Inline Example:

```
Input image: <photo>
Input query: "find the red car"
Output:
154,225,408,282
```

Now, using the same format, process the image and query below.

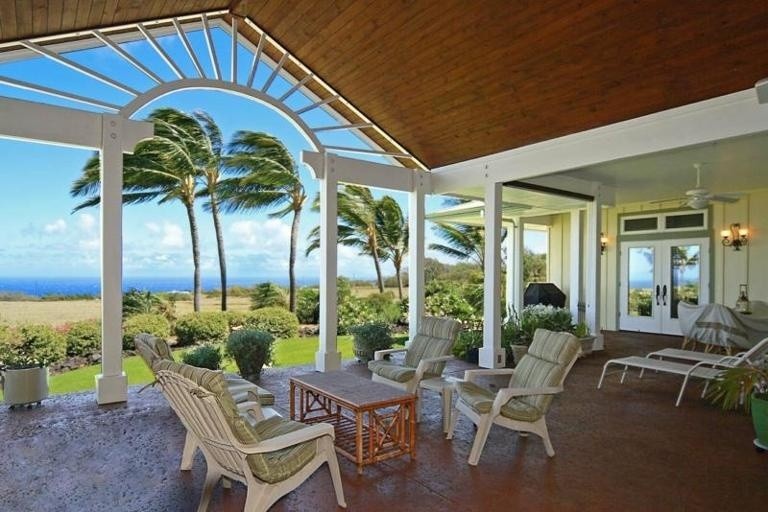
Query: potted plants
2,325,51,410
459,331,483,364
347,319,390,364
701,364,768,454
223,330,273,382
575,324,595,355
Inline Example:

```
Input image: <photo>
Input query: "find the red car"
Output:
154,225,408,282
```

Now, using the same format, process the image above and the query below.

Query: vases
510,345,528,365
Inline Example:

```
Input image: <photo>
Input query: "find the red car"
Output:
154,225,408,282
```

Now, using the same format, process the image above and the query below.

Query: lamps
600,233,607,255
686,199,705,209
720,223,749,251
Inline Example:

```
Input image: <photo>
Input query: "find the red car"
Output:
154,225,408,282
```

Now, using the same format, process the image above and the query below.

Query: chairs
597,336,768,408
446,327,583,466
677,301,732,355
133,332,348,512
367,316,462,433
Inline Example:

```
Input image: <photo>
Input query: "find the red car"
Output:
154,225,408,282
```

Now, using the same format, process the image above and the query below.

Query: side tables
416,374,463,433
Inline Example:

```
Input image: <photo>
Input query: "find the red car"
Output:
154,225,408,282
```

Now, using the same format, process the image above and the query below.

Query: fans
649,163,740,209
